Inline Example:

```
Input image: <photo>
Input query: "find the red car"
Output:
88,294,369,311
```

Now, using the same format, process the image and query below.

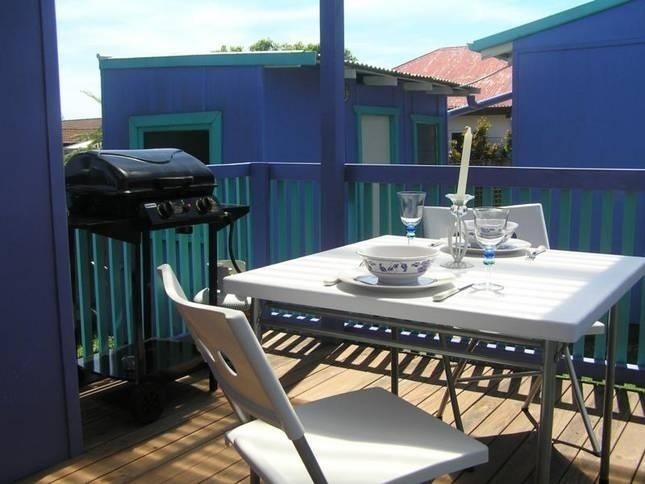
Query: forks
524,245,546,266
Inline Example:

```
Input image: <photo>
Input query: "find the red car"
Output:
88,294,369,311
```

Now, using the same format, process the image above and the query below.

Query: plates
338,268,457,294
446,236,530,255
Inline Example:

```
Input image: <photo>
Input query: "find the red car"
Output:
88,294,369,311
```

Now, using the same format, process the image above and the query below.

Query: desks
68,204,250,425
222,234,645,484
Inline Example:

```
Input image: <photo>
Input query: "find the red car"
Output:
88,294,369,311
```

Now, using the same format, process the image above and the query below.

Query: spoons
323,259,370,287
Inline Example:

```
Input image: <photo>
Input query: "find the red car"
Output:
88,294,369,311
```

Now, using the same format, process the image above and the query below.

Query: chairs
417,204,609,456
157,254,492,484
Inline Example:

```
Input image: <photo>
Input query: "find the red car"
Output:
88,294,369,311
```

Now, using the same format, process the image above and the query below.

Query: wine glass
396,190,427,248
474,206,510,292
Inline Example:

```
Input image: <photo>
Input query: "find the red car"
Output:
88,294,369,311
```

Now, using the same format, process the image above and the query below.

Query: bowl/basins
454,220,519,246
353,245,440,283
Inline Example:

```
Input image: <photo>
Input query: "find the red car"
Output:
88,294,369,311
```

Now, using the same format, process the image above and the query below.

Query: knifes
429,280,479,302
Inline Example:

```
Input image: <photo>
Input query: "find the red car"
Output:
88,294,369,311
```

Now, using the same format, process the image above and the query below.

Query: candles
458,121,474,194
